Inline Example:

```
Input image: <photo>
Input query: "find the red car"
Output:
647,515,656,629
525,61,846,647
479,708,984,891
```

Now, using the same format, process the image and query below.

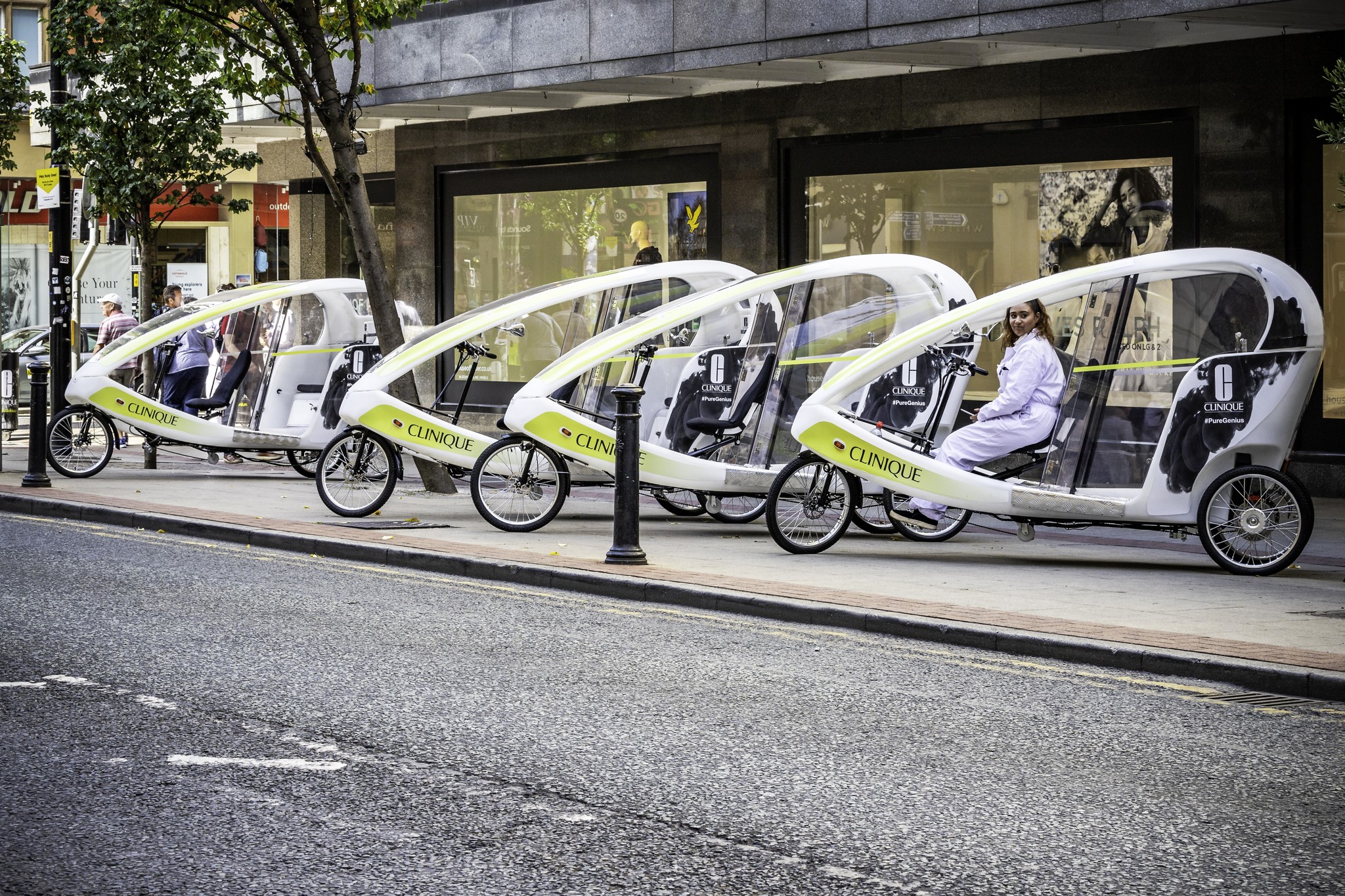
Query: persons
614,220,663,387
1042,167,1173,396
887,297,1064,530
92,292,142,448
459,290,597,385
150,283,297,463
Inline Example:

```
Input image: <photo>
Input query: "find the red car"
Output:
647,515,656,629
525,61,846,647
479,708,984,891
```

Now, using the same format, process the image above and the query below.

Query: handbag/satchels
215,335,225,355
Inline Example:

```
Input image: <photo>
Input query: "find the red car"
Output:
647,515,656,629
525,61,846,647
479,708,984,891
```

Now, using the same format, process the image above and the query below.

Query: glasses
1044,261,1060,275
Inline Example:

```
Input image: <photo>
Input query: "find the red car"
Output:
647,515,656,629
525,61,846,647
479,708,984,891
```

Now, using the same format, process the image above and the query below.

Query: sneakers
122,436,128,445
890,508,938,531
113,438,126,448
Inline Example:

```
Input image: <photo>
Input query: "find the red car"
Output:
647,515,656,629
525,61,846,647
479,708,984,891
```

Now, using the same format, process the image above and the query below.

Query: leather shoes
256,451,284,460
223,454,243,463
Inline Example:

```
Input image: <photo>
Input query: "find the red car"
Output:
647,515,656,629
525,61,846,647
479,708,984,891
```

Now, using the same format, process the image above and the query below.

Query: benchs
297,382,325,394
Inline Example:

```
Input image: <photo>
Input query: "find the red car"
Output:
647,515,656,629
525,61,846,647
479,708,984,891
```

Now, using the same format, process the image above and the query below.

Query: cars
1,322,106,406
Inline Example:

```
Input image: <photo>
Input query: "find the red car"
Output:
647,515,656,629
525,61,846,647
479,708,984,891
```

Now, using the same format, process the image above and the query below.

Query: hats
96,293,122,306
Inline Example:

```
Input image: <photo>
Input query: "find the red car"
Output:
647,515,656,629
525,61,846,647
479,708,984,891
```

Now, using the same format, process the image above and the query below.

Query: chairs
1005,358,1101,464
185,348,252,418
685,350,777,444
496,369,581,432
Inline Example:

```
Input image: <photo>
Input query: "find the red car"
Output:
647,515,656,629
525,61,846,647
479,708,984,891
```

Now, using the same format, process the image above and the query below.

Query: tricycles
471,251,982,541
763,245,1326,576
312,257,784,520
47,275,422,484
